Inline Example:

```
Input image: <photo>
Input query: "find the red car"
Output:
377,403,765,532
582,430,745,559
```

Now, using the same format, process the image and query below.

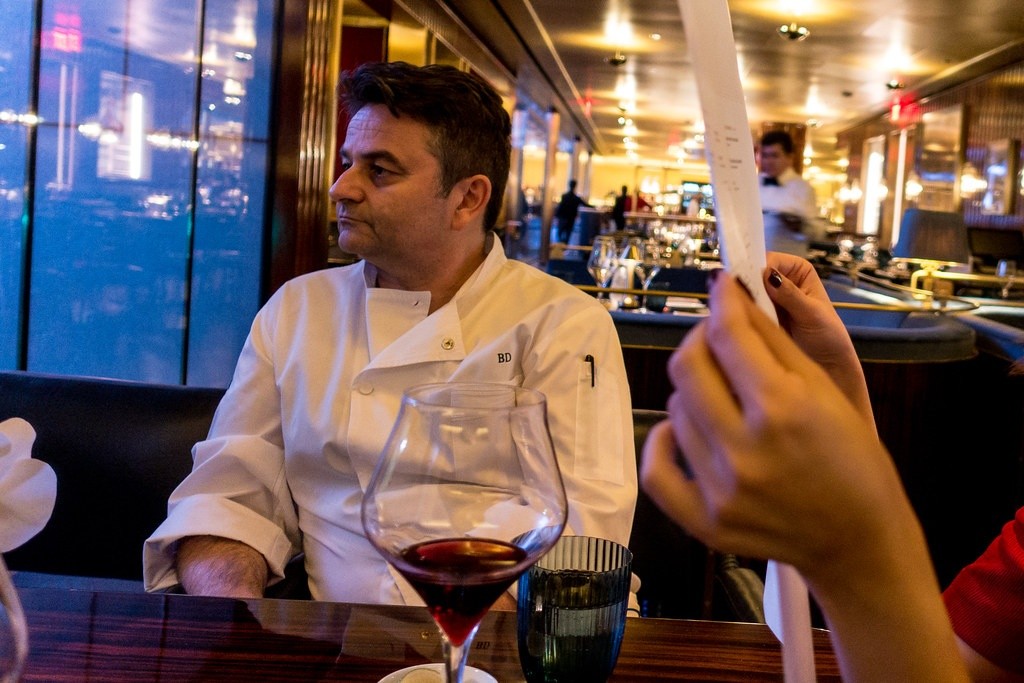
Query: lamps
893,207,970,296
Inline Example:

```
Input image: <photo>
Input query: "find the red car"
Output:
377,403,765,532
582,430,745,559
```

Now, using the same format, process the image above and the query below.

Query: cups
517,535,632,683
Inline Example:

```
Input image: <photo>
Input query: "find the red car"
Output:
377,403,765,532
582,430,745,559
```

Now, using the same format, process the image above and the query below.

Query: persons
757,131,818,259
555,180,595,245
143,61,640,618
640,252,1024,683
612,186,652,230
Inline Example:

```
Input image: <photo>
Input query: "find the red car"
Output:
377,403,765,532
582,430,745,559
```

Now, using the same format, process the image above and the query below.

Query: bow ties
763,175,779,186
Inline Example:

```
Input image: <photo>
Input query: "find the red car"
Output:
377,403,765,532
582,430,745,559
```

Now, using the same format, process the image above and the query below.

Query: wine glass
587,236,618,300
362,382,570,683
996,261,1015,297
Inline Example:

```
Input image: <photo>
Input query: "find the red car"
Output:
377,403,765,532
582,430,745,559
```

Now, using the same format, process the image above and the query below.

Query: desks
0,571,845,681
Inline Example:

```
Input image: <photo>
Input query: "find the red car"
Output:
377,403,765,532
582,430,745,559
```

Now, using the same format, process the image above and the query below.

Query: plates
373,662,496,683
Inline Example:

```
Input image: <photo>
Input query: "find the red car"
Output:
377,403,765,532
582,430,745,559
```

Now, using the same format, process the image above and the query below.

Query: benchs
551,212,1024,623
